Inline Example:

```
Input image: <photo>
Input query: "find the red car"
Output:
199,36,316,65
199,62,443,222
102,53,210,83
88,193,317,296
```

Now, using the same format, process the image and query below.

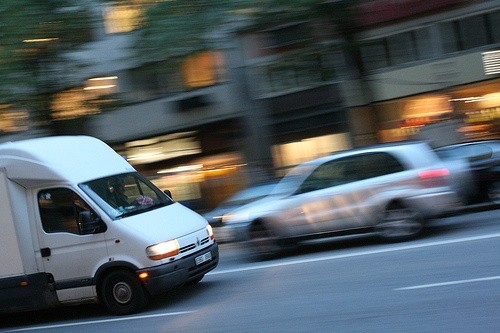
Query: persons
109,179,131,211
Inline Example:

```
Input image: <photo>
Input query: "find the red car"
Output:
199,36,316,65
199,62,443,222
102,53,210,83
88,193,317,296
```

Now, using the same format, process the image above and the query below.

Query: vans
0,134,220,315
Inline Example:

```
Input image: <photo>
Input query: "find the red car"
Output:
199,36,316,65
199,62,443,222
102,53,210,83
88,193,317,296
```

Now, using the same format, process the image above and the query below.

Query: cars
224,141,469,258
201,174,298,244
429,136,500,218
